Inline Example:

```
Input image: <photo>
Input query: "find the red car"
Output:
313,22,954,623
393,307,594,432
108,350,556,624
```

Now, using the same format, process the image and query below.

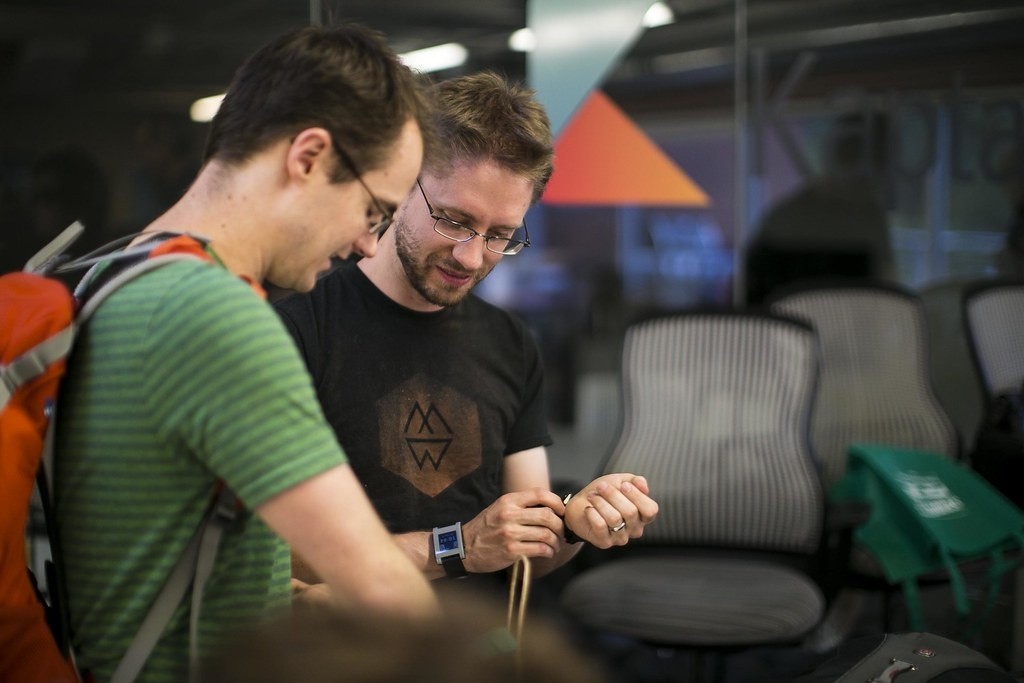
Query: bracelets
560,492,587,544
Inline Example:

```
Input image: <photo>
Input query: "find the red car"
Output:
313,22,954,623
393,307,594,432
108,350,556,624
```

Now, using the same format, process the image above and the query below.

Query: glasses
328,134,392,231
416,178,531,256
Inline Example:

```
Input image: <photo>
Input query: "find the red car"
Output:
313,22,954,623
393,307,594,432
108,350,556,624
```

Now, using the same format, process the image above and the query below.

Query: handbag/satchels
839,439,1024,635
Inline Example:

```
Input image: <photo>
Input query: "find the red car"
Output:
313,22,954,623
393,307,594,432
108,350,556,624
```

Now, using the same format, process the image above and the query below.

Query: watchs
431,520,478,576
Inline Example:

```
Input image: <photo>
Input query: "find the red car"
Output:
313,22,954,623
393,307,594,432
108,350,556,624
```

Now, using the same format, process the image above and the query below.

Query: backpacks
0,222,267,682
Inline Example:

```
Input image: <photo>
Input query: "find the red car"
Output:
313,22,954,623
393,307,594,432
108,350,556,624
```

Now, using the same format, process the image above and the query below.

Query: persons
270,66,660,683
51,23,445,683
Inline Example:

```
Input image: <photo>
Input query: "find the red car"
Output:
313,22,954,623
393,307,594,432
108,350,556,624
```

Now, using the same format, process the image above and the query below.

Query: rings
609,521,626,532
